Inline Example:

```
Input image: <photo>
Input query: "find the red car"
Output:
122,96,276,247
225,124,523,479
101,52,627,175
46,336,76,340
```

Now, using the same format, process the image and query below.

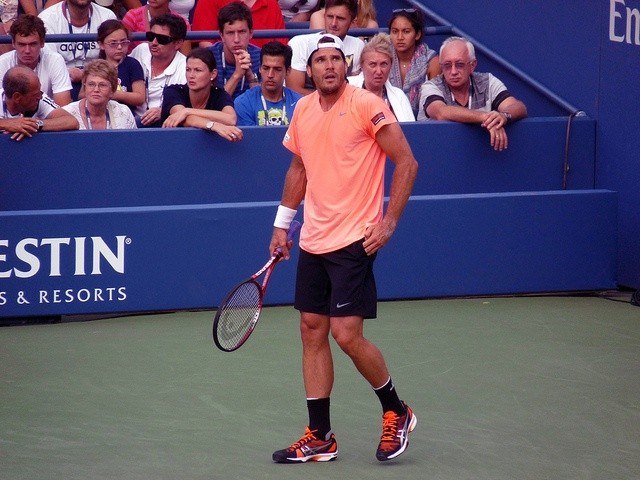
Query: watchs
36,119,44,131
503,111,511,122
206,121,214,130
248,72,259,83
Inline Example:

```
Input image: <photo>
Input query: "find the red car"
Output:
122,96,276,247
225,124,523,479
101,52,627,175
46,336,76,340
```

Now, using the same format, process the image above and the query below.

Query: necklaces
400,63,410,67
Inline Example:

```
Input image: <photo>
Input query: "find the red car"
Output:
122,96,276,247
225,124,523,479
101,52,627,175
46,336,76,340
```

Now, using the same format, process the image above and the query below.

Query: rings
231,133,235,137
496,135,499,138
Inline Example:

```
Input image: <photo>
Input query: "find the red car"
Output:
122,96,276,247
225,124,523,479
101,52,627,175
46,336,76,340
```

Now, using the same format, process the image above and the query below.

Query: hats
305,31,345,64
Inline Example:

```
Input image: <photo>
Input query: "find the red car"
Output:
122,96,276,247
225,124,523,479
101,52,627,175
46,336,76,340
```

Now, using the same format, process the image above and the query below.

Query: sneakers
272,426,338,463
376,400,417,461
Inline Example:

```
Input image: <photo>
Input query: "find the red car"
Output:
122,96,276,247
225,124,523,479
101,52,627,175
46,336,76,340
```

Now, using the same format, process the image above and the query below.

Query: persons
169,0,196,22
78,18,146,116
192,0,289,49
283,0,366,96
388,7,442,121
206,0,261,100
0,0,38,56
278,0,318,21
160,47,244,142
0,64,80,141
43,0,143,19
348,33,416,122
127,13,188,128
417,36,528,150
37,0,117,98
122,0,191,55
310,0,379,44
235,40,304,126
0,13,74,107
269,33,419,465
62,58,138,131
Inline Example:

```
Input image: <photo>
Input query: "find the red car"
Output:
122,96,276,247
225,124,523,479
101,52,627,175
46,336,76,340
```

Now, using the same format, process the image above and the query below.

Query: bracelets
273,205,297,229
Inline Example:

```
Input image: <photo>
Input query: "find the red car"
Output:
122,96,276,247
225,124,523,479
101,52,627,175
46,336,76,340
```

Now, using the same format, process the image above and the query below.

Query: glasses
439,61,473,68
392,8,417,13
103,40,130,48
146,32,178,45
82,82,112,89
